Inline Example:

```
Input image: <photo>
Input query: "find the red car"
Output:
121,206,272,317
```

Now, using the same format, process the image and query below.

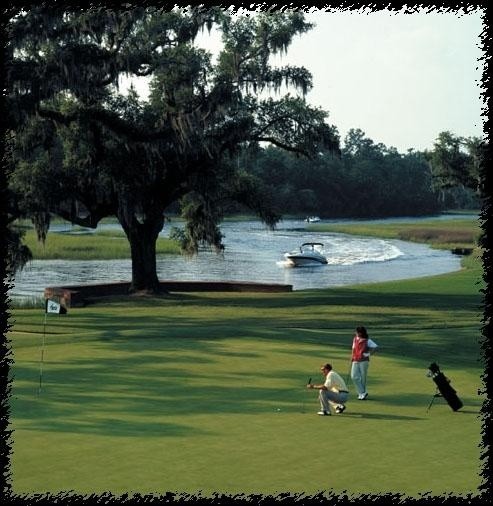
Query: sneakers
358,393,368,400
336,406,344,414
317,411,330,416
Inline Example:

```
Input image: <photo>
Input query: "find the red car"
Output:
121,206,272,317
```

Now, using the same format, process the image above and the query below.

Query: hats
321,364,331,369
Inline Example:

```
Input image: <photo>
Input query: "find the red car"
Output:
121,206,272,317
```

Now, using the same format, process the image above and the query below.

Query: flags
47,300,67,314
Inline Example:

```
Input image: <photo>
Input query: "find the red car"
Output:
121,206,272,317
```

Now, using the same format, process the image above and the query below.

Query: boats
304,215,320,222
285,240,328,265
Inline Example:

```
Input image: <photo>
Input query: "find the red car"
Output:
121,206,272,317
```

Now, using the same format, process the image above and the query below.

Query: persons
351,326,378,400
307,364,349,416
